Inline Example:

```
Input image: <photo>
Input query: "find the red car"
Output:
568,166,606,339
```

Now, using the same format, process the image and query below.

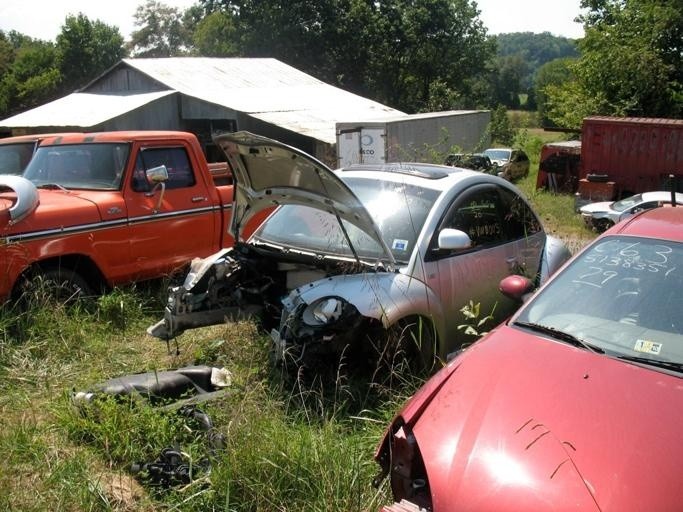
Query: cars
362,193,683,511
0,134,78,178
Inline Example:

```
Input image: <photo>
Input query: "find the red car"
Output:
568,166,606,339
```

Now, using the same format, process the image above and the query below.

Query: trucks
1,125,288,336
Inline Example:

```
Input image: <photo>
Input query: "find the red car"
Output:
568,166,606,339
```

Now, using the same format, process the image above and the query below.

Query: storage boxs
578,178,616,200
